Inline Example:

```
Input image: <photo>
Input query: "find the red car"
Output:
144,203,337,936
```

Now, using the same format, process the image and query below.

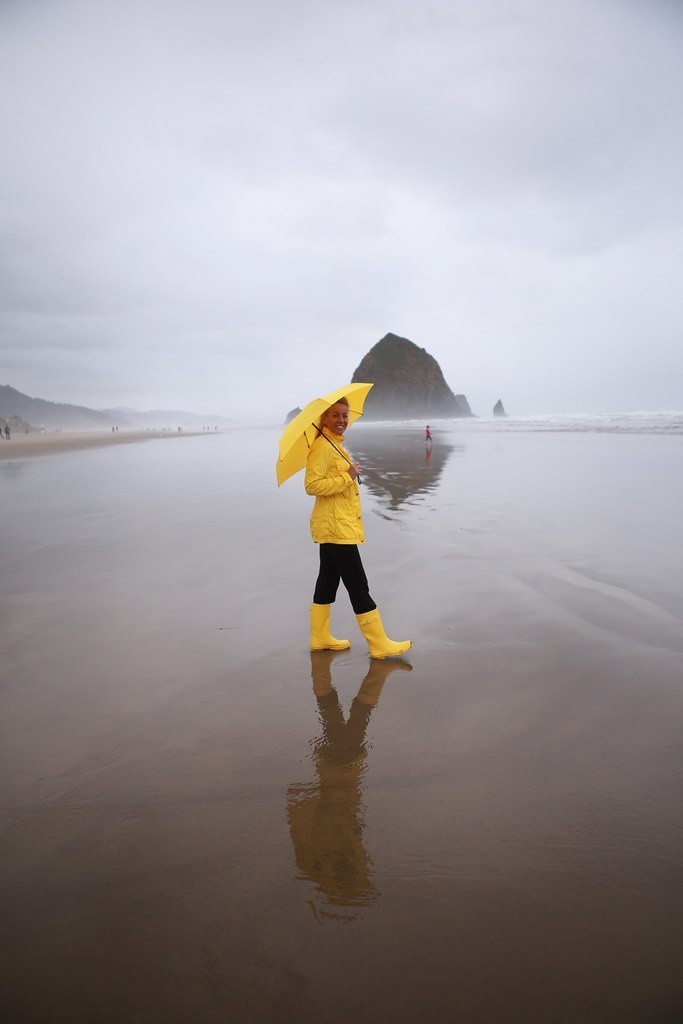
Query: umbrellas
276,383,375,487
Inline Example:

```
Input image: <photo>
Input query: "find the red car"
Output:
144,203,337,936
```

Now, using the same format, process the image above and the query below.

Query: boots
310,648,349,696
309,603,350,651
356,607,412,659
356,659,413,706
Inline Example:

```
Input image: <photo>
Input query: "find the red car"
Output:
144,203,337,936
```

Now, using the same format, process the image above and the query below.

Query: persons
5,424,10,440
0,429,4,439
304,399,414,660
425,426,431,440
